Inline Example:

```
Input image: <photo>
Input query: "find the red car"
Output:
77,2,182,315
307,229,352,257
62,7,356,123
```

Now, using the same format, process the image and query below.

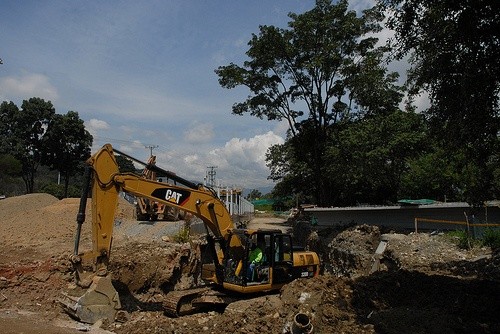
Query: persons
199,184,205,191
158,178,163,182
196,185,199,190
244,243,263,282
191,183,195,188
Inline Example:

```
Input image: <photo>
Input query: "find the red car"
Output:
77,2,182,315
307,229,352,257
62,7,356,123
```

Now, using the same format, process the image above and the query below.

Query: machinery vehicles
54,143,322,326
